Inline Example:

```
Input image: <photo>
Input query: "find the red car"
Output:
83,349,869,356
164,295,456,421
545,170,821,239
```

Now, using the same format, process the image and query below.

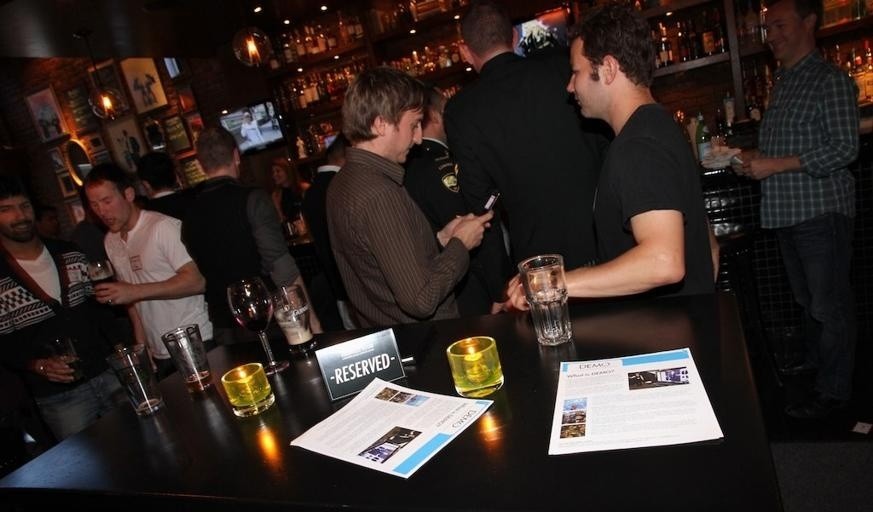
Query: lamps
228,0,274,69
71,32,126,121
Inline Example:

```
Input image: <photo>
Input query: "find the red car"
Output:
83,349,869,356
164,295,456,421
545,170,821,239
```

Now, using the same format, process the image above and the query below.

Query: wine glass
226,278,290,377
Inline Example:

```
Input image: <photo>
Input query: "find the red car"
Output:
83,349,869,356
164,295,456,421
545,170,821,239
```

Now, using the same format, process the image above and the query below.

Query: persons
732,0,861,419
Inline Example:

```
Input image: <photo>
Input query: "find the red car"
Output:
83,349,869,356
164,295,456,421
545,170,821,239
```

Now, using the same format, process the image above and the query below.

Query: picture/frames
117,55,170,118
21,80,113,230
100,113,149,175
84,58,133,115
162,82,209,187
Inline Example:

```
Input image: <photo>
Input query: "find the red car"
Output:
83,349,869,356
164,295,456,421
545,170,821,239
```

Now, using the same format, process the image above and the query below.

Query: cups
85,262,116,293
161,323,211,392
221,363,275,419
268,285,317,354
106,343,162,417
50,338,80,372
446,336,503,399
519,254,571,345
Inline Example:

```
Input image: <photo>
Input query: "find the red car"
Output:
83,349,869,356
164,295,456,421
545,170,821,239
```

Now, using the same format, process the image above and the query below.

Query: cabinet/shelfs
655,2,868,164
261,0,471,165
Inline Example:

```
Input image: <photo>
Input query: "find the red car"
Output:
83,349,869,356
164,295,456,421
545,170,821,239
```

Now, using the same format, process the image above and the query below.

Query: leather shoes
784,389,850,421
779,359,817,379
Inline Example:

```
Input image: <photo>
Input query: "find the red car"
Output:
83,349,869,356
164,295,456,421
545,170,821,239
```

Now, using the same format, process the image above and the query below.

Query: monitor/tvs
216,99,290,158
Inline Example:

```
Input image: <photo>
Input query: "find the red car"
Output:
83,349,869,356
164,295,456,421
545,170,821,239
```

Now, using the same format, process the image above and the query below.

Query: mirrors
140,119,168,155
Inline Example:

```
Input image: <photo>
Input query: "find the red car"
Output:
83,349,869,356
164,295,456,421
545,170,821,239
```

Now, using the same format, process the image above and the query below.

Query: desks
0,292,789,512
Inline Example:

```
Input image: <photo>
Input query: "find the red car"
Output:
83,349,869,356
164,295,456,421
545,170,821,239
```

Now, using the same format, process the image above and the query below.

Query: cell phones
475,190,500,217
730,155,744,165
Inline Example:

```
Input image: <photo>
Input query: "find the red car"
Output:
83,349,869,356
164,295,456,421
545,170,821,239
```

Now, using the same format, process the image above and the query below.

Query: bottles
645,0,873,167
248,2,476,160
